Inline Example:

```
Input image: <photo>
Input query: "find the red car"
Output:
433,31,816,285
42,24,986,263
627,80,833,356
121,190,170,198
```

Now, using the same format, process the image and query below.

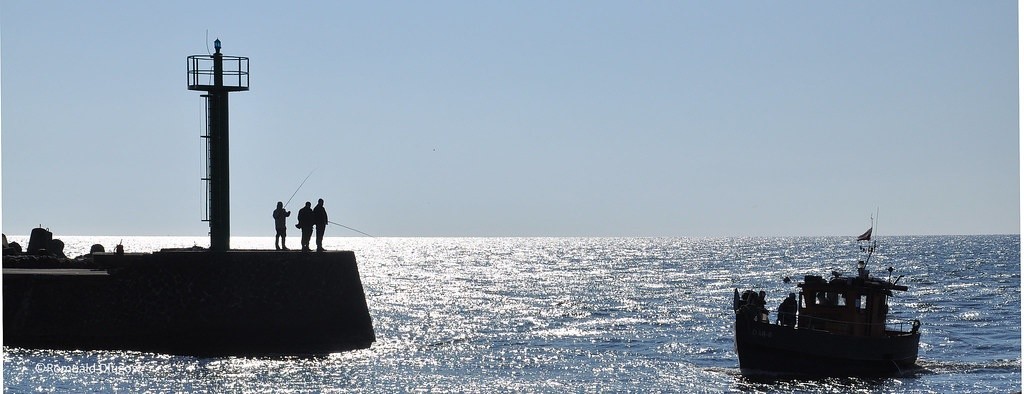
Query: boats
732,244,922,385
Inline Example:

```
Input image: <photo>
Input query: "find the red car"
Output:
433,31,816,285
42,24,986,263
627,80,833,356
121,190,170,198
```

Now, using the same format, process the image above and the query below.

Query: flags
858,227,873,242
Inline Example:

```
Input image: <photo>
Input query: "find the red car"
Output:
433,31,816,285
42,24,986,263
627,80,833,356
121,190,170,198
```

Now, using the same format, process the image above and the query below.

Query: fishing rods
328,221,381,242
283,167,316,209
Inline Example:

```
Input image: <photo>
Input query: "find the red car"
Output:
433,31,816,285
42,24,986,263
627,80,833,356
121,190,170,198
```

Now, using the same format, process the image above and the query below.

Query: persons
778,293,797,329
298,202,313,251
313,199,328,251
816,293,830,305
273,201,290,250
752,290,769,320
